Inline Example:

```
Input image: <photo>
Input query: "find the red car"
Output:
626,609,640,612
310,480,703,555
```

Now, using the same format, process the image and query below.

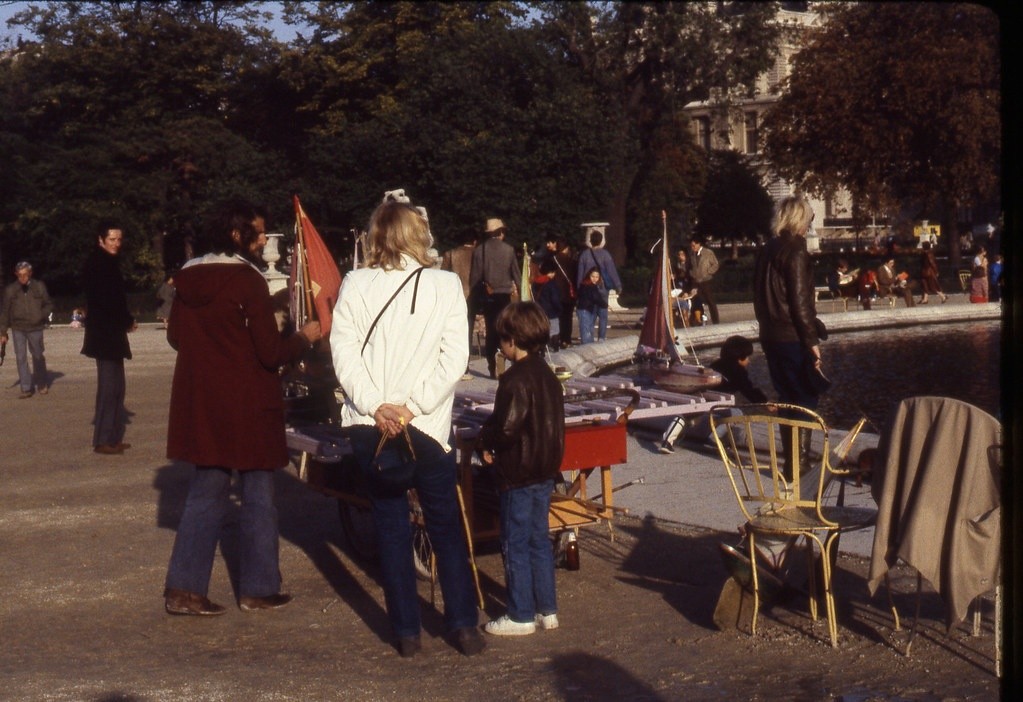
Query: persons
442,226,482,379
532,234,578,347
165,198,322,617
81,221,138,454
702,336,776,449
753,197,828,483
328,201,486,658
69,309,84,328
470,218,521,377
576,266,609,343
949,230,1002,303
915,241,948,304
156,276,176,328
672,236,720,324
828,257,916,310
475,301,566,636
0,261,53,398
577,231,622,343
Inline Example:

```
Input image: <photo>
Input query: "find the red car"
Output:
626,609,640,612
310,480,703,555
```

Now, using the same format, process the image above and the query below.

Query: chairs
708,402,879,649
867,396,1001,638
958,269,972,302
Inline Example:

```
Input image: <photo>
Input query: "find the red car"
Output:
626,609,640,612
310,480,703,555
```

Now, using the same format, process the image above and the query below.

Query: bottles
494,346,505,379
566,533,580,571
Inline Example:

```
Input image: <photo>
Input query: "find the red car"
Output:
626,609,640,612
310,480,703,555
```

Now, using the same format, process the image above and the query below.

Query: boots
779,427,795,482
798,427,813,477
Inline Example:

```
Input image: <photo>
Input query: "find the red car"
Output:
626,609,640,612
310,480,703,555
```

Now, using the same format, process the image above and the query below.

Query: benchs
825,275,899,313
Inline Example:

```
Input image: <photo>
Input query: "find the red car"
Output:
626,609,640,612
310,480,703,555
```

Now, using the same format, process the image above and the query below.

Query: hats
484,219,507,231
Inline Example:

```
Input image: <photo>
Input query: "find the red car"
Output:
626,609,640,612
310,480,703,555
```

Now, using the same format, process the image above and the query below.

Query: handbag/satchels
568,279,575,297
465,280,495,315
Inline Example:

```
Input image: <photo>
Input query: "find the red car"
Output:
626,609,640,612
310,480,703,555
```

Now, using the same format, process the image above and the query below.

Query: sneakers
533,612,558,629
485,614,535,636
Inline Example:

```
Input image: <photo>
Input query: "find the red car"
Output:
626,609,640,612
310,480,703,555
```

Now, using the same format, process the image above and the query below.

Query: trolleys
226,378,641,581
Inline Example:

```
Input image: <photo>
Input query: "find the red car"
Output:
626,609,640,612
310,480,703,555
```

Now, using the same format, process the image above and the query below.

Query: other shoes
488,365,496,378
165,589,227,614
400,633,421,656
941,295,949,303
118,443,131,449
38,380,48,393
660,441,674,453
918,300,928,304
19,389,34,399
462,373,475,380
239,592,294,610
94,445,123,454
455,627,484,655
495,352,506,377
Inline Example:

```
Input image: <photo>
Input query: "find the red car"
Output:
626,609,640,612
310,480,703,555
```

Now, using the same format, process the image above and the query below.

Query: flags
288,195,343,384
